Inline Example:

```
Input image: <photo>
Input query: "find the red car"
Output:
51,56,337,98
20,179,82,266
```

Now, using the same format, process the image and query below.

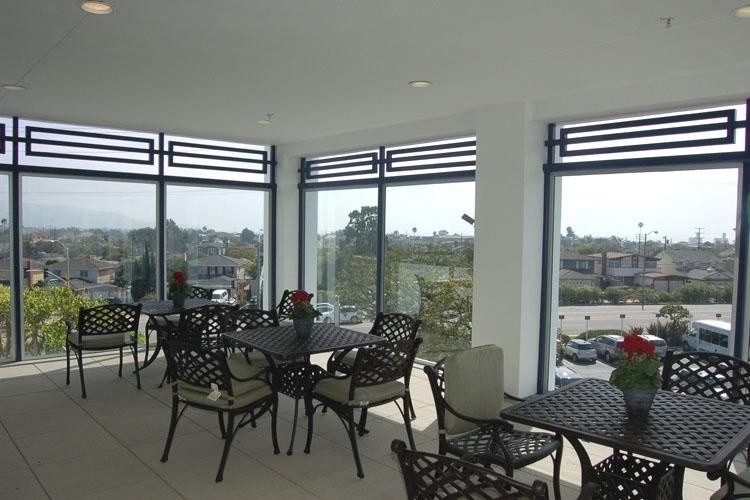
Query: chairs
391,437,551,500
660,349,750,406
63,301,142,400
706,457,750,499
423,343,563,500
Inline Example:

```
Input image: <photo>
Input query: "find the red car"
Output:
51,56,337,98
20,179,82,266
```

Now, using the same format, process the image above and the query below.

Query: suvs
589,335,627,362
556,340,564,364
564,339,599,364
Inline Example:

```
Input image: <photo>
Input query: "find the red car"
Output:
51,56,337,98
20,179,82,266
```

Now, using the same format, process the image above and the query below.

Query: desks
498,374,749,500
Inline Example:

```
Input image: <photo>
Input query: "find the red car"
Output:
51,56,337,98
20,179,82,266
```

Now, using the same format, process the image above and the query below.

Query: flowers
606,334,663,391
169,270,189,295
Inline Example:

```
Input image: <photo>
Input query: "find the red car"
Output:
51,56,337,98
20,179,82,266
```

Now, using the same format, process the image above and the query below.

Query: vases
624,388,654,421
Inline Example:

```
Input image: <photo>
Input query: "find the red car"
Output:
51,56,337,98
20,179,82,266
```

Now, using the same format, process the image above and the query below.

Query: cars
320,305,367,325
316,303,333,319
249,295,257,303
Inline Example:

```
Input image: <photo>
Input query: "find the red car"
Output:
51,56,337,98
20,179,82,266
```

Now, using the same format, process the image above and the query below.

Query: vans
211,289,228,305
637,336,668,359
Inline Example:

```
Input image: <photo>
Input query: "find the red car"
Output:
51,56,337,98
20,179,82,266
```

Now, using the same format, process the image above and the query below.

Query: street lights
257,227,264,277
558,314,565,340
48,239,70,291
620,314,626,334
637,222,643,253
716,313,722,321
412,227,417,248
585,315,590,341
642,231,659,311
655,314,661,338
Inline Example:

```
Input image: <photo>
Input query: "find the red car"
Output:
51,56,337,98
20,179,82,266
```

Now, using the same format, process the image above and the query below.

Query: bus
681,319,732,356
